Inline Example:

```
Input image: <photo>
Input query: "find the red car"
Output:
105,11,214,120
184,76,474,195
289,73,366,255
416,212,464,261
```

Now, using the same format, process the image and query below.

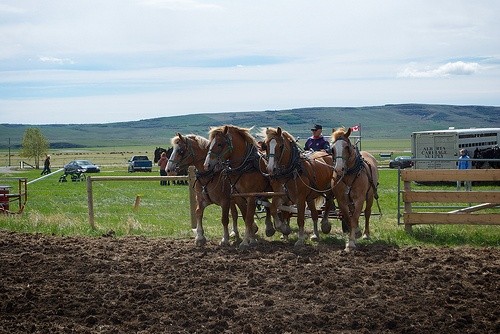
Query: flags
350,124,360,131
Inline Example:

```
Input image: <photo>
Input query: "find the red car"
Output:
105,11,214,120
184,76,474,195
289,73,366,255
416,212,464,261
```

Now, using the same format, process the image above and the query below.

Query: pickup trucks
127,154,152,173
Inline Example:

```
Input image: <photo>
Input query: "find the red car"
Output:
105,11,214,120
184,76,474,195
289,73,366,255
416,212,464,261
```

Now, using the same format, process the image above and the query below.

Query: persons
304,125,331,154
158,152,169,186
41,156,52,175
455,148,472,191
473,147,480,159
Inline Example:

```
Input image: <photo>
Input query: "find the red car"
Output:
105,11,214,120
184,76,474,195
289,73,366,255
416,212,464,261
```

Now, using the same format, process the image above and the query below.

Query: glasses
312,129,317,131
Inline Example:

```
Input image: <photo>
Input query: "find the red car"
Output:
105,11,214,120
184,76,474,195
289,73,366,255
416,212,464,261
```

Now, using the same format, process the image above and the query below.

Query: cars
389,155,413,170
63,159,100,175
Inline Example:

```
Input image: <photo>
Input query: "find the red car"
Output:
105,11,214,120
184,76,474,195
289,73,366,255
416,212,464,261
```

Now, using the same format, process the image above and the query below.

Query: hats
311,125,322,130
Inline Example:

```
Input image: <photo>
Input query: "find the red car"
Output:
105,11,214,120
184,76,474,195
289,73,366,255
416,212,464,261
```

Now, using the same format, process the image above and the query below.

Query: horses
164,125,379,253
